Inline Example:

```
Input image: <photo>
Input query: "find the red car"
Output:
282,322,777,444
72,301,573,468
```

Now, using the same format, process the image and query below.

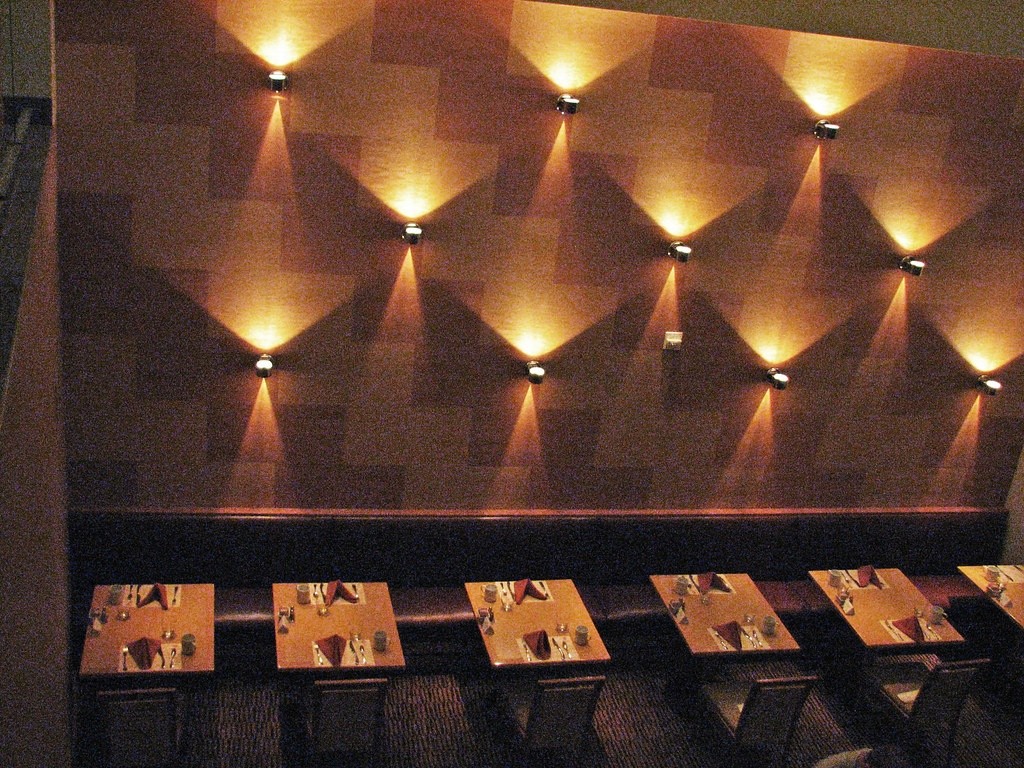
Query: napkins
712,620,748,650
515,578,547,604
522,629,552,660
317,633,347,667
893,614,926,642
127,638,161,669
697,571,731,596
325,579,357,605
858,565,881,588
137,584,169,610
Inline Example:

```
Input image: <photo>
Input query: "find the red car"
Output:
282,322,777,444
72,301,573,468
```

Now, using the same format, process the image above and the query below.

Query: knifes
846,570,861,588
320,583,326,602
742,627,757,648
136,584,141,605
552,638,565,660
348,641,359,665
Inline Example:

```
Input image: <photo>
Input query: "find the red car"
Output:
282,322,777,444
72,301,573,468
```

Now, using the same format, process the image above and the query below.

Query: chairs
503,673,607,768
862,657,993,768
94,687,191,768
300,676,389,768
695,674,821,768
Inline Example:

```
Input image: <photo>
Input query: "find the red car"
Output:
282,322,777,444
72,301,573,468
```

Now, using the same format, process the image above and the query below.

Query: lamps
524,360,546,385
814,118,843,141
667,241,693,264
557,92,583,115
976,372,1002,397
254,353,275,379
899,255,926,277
766,367,789,391
267,70,290,93
398,220,426,246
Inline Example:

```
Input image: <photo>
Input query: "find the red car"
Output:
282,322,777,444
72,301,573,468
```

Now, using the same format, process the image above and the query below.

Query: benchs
66,506,1009,674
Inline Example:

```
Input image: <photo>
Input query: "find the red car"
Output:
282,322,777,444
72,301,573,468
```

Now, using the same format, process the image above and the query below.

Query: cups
181,633,196,655
374,629,391,652
575,625,592,645
828,570,843,588
555,617,568,632
985,566,1000,582
161,618,175,638
674,576,688,594
929,606,948,624
296,584,311,604
118,602,130,619
501,597,514,611
481,584,497,603
316,601,329,614
762,616,781,635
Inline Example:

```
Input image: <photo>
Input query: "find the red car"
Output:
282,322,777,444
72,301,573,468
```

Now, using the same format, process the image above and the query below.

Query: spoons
312,584,319,598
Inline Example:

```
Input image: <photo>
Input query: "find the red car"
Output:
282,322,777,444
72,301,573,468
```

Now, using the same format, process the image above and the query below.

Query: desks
78,583,217,679
464,578,610,719
270,581,406,679
806,564,965,700
955,562,1024,633
649,571,801,697
